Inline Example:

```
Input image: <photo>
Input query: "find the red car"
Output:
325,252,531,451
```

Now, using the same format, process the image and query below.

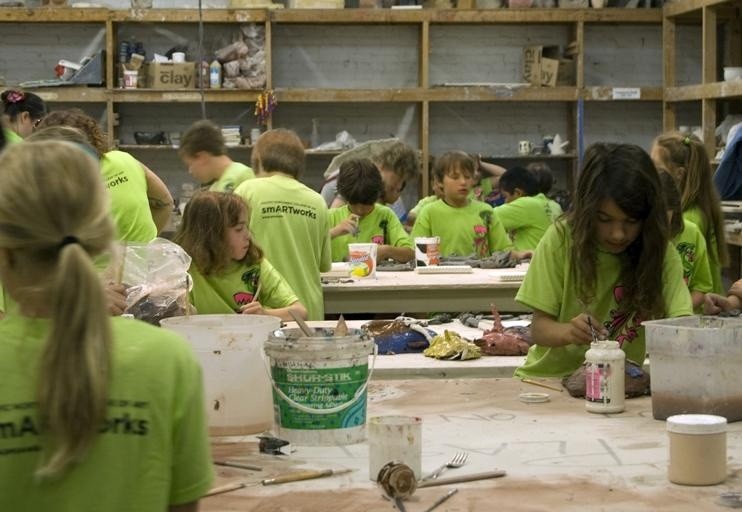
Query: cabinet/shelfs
661,0,742,246
0,0,722,211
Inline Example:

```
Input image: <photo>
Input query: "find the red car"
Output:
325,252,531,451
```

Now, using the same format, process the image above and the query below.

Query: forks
419,453,468,483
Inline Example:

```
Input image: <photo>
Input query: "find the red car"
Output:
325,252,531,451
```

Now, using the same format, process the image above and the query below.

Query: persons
0,140,217,512
512,126,742,377
323,138,565,264
0,92,331,323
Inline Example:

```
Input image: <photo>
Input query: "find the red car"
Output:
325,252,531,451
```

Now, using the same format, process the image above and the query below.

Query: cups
348,244,378,280
123,71,137,88
519,140,535,155
415,238,441,269
121,41,144,62
368,416,423,483
171,53,185,63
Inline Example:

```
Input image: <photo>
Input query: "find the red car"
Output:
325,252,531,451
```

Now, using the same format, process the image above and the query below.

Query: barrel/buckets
158,313,281,437
265,327,378,448
640,313,742,424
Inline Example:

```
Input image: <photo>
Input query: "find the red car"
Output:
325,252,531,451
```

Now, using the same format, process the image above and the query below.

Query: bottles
586,342,626,414
668,415,729,485
199,61,210,88
209,57,221,87
251,127,260,145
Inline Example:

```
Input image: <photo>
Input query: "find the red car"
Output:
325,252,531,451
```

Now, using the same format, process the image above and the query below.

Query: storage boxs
146,62,198,91
521,41,577,88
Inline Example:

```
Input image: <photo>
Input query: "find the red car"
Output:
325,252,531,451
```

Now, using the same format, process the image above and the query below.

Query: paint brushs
261,467,356,486
213,458,263,471
424,487,459,511
249,283,262,305
521,378,563,392
356,217,359,243
200,483,245,496
417,468,507,488
117,238,128,285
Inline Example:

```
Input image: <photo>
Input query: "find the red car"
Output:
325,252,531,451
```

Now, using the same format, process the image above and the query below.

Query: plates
520,393,550,404
